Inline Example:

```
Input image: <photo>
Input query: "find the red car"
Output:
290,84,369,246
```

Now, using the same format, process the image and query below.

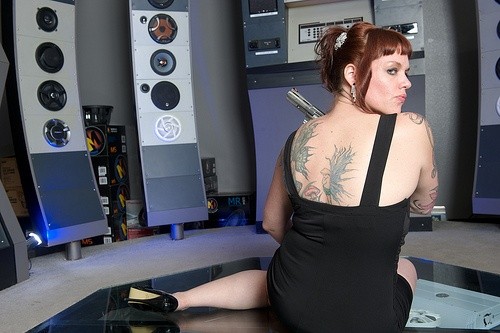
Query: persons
124,22,439,333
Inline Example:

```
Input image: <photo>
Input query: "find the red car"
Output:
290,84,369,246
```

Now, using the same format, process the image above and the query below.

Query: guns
286,88,326,125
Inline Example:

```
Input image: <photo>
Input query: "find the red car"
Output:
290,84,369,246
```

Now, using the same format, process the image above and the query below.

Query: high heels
110,286,178,312
